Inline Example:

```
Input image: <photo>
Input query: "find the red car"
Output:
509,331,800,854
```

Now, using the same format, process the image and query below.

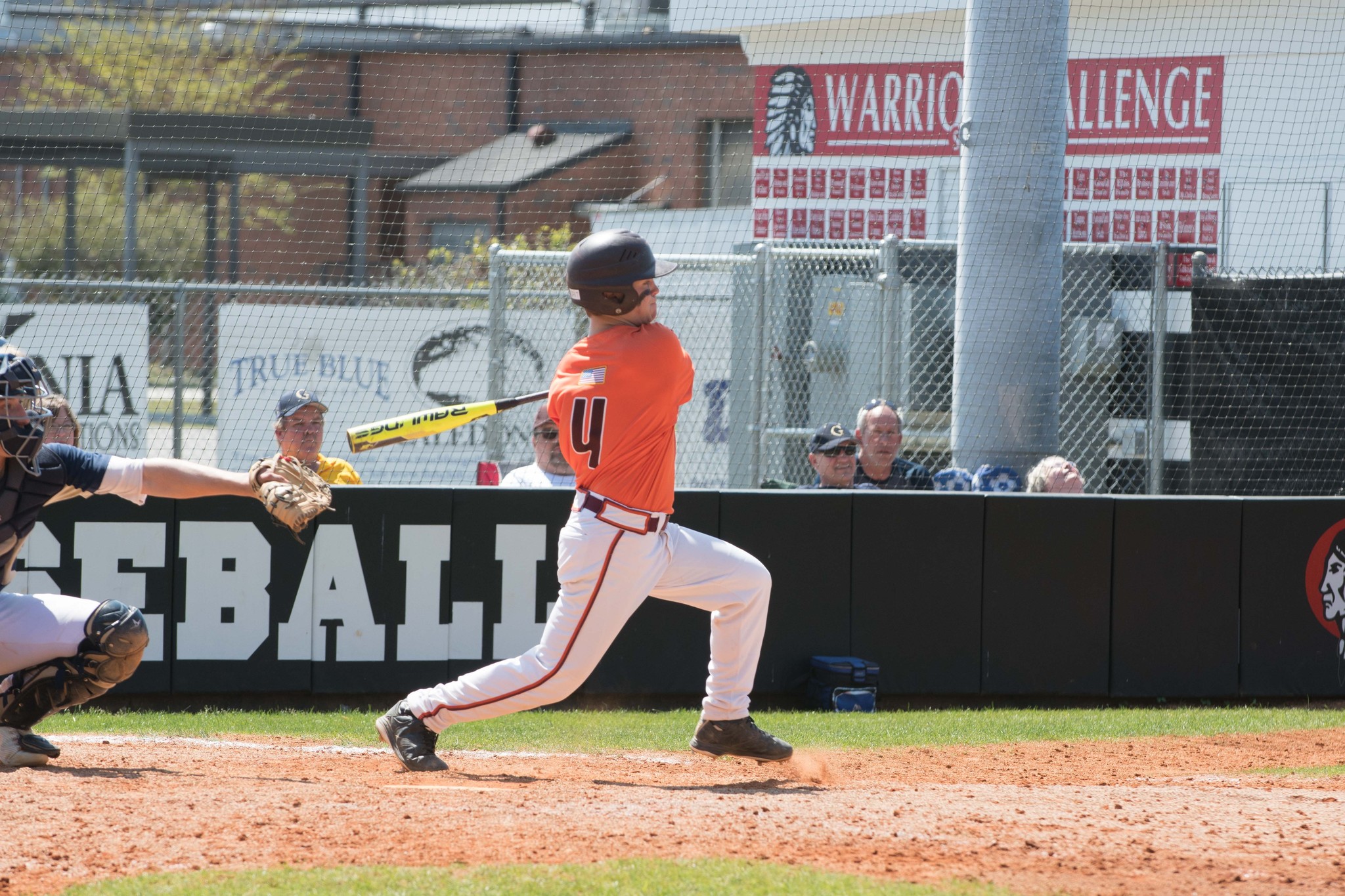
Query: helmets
0,336,52,476
567,230,678,316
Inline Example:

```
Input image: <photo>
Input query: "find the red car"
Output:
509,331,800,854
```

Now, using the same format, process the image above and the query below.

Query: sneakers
0,726,48,769
690,717,792,766
376,700,449,772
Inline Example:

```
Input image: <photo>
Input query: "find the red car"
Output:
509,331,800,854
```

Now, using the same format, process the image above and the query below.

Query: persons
0,328,332,767
34,394,79,447
793,422,880,489
274,389,362,485
498,404,577,490
377,228,793,772
853,399,936,490
1025,455,1085,493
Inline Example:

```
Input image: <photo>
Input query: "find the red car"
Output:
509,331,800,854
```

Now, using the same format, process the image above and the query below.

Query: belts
582,495,670,531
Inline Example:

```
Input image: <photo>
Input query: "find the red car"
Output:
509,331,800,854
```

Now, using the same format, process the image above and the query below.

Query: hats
533,405,551,428
276,390,327,420
808,423,861,453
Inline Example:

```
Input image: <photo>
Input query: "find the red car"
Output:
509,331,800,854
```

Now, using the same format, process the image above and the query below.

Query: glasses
864,399,898,411
823,445,856,457
534,428,559,438
1044,462,1076,481
49,421,76,433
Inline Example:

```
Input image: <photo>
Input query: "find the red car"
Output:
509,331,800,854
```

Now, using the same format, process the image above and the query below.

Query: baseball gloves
248,452,337,546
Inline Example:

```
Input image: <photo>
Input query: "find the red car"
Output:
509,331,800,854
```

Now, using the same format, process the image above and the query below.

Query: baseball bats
346,388,551,455
